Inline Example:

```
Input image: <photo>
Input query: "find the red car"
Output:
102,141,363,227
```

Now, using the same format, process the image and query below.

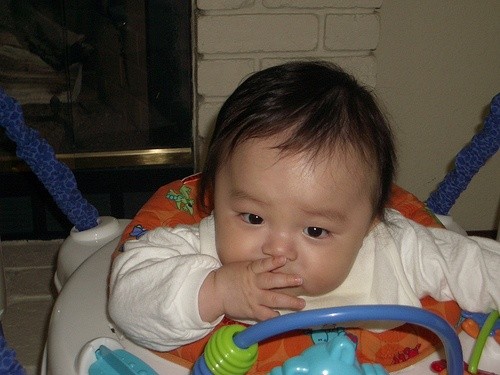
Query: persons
108,59,500,374
